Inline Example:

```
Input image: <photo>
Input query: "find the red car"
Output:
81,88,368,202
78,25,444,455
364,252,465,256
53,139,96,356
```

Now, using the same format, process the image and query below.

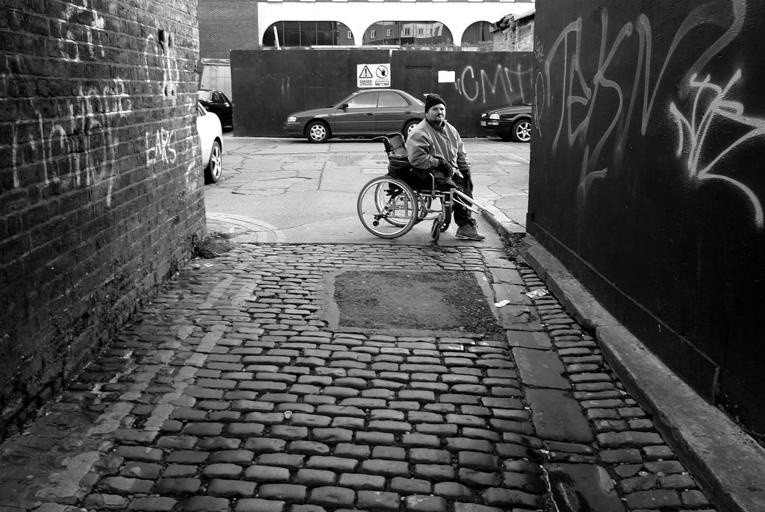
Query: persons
405,92,484,241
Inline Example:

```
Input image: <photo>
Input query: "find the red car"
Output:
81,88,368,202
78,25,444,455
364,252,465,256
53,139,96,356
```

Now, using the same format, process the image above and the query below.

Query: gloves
463,176,473,193
438,159,453,177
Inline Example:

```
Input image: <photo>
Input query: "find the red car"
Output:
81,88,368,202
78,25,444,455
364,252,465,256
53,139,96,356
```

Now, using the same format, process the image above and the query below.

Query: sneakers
455,217,485,241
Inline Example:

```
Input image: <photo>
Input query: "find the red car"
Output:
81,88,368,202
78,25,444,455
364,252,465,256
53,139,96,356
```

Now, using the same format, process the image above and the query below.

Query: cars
197,86,232,183
481,100,532,143
284,88,439,144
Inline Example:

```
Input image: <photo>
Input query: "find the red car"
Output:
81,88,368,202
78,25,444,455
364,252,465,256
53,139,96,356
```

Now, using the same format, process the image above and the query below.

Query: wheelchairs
356,132,453,240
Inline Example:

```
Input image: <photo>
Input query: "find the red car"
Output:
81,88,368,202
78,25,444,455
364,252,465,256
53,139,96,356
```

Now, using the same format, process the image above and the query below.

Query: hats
425,94,445,112
